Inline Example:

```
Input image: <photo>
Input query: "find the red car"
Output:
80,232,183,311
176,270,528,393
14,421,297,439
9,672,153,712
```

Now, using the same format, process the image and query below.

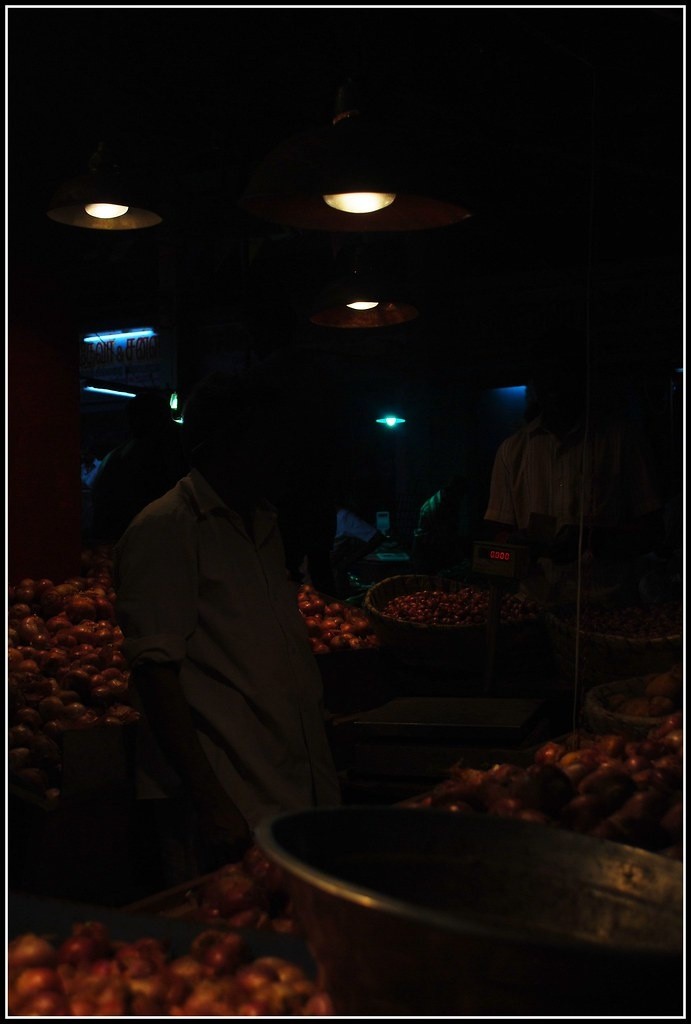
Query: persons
108,360,347,905
482,324,670,610
413,472,470,578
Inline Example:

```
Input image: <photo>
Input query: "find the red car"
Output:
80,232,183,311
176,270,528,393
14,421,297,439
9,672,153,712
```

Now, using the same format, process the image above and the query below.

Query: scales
350,693,556,779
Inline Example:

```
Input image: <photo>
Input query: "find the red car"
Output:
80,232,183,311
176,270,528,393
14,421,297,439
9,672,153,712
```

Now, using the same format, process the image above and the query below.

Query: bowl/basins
252,803,683,1017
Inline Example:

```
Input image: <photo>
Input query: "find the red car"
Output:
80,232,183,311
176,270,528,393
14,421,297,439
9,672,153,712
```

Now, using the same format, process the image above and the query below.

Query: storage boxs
60,572,682,1016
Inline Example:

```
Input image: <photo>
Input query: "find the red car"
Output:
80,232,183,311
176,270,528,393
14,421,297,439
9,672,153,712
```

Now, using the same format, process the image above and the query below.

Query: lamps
307,233,421,330
238,9,474,236
41,13,168,234
